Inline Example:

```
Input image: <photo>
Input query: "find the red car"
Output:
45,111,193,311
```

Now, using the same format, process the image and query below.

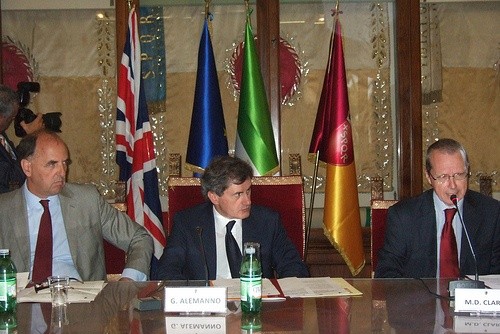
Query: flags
115,6,167,279
309,19,365,276
235,20,280,176
185,20,229,178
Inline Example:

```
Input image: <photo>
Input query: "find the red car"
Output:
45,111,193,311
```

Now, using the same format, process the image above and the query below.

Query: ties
225,220,242,278
32,200,53,282
440,208,460,278
439,278,459,330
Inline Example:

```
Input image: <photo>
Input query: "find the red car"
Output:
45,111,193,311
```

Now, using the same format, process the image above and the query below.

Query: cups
47,276,70,308
238,242,262,277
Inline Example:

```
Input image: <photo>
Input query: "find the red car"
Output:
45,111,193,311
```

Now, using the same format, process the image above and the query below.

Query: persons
0,85,46,193
375,138,500,278
159,157,309,279
14,277,469,334
0,129,154,284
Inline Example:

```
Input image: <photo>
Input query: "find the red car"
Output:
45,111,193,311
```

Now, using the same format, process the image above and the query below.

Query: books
16,283,108,302
210,278,363,299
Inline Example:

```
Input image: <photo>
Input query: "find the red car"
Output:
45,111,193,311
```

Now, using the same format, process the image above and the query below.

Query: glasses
430,170,467,184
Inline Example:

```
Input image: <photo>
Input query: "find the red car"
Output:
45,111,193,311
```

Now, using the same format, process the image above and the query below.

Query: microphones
196,226,209,286
449,193,485,297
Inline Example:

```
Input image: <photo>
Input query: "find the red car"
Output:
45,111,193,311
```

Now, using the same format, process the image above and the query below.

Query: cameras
14,81,62,133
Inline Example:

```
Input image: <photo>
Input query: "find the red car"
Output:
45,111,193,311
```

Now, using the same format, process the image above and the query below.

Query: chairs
370,176,493,279
167,153,306,260
9,183,131,274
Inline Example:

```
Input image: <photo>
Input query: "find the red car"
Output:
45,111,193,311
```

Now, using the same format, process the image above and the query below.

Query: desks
14,274,500,334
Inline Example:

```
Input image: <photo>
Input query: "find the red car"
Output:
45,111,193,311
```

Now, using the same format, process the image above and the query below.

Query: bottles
239,248,262,313
0,249,17,316
